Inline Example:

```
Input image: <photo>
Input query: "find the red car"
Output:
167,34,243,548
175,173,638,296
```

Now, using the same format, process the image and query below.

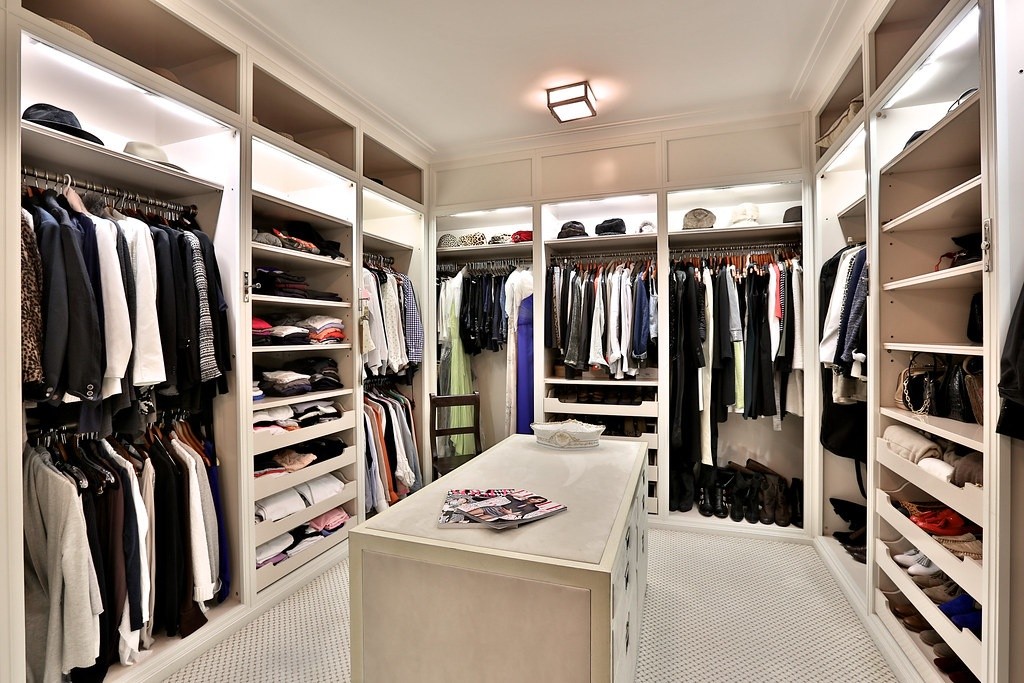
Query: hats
123,142,188,173
23,103,104,146
438,233,459,247
595,218,626,235
488,233,512,244
682,208,716,229
512,231,532,243
727,204,760,227
782,206,802,223
460,232,487,246
557,221,589,238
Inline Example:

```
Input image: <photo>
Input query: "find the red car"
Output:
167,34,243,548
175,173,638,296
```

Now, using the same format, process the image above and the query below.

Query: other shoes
544,383,657,437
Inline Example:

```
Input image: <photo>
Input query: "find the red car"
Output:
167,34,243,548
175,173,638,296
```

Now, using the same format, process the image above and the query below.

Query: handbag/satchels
894,352,983,426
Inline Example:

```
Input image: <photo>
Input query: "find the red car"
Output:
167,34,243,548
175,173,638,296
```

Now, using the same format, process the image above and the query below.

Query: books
456,487,567,530
436,487,518,529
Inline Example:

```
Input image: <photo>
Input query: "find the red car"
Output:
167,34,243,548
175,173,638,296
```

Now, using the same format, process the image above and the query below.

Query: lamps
546,80,597,123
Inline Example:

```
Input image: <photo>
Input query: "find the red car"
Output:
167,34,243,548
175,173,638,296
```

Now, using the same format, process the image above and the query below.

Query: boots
670,456,803,527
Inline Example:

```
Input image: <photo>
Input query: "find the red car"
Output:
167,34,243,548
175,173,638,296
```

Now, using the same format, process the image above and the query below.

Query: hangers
362,253,394,275
670,242,802,280
548,250,657,281
24,406,212,489
21,165,209,240
435,255,526,281
364,378,411,416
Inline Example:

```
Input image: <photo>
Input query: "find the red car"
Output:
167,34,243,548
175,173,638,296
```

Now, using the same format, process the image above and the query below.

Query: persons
467,494,548,521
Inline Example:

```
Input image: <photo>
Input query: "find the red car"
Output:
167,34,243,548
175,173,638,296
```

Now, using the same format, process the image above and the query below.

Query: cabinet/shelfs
348,434,647,683
0,0,250,683
662,172,809,544
360,118,432,523
810,18,869,635
865,0,1024,683
541,192,657,515
428,201,537,486
247,52,360,610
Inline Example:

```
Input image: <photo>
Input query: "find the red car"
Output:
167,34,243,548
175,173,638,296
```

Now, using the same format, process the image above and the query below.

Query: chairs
430,390,482,482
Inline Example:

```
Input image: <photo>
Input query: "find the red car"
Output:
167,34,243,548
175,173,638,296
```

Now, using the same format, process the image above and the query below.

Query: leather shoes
881,499,984,683
829,497,866,564
